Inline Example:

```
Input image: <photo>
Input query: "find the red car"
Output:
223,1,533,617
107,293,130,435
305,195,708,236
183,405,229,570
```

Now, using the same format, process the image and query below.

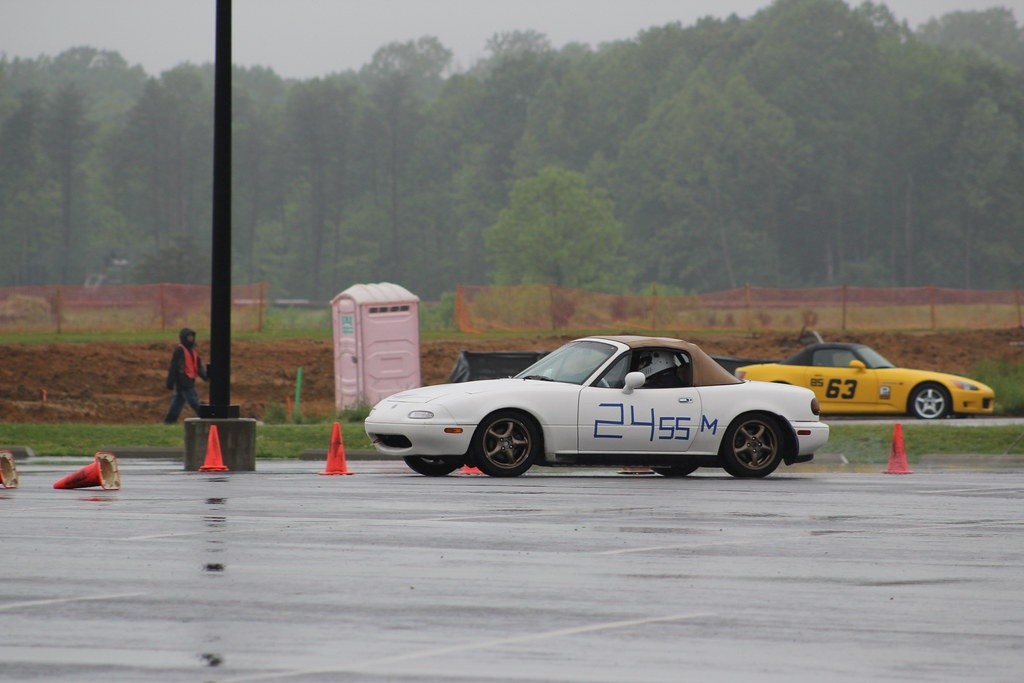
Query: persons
161,328,209,424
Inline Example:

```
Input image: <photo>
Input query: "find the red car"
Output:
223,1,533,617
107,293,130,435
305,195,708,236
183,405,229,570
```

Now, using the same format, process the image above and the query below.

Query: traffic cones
52,451,122,494
459,463,485,476
880,423,915,476
0,448,20,489
197,424,230,473
317,421,355,476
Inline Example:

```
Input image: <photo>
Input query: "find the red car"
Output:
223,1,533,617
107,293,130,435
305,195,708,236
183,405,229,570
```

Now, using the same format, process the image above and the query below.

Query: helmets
639,349,675,378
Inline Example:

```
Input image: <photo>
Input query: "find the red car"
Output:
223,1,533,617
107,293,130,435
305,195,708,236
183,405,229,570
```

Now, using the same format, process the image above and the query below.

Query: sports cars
733,341,998,422
358,336,832,481
